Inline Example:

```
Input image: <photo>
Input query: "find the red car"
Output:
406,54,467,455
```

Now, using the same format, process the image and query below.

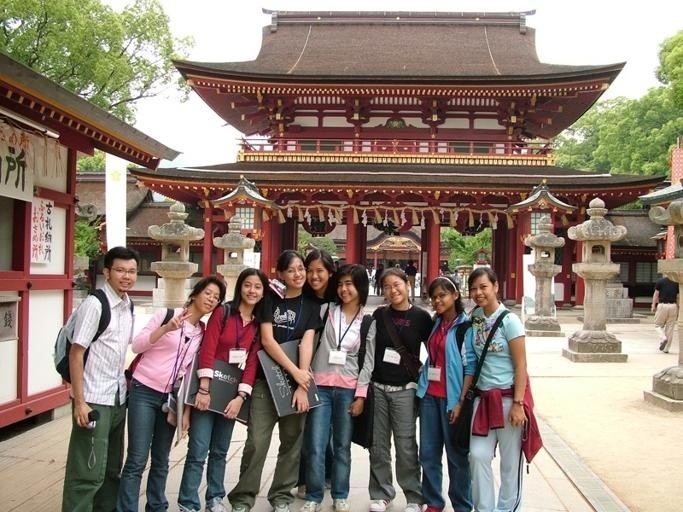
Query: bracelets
198,391,210,395
198,388,210,392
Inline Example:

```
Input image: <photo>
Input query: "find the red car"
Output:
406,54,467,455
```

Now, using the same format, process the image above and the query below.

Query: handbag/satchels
450,390,475,449
351,382,374,448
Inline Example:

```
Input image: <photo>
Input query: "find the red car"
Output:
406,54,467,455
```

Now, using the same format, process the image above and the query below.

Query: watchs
513,400,524,406
237,394,247,401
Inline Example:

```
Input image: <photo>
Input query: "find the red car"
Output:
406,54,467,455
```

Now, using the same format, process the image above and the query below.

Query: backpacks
124,308,204,409
54,289,133,384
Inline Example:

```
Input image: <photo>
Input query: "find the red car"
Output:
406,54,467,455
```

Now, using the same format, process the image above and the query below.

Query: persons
227,249,324,512
298,264,378,512
293,249,342,499
335,259,462,301
650,277,679,353
468,267,543,512
178,268,269,512
115,277,226,512
54,246,138,512
415,275,478,512
368,266,434,512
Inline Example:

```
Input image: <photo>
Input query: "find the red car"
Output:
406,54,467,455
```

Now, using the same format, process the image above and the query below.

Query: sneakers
206,496,230,512
333,499,349,512
231,504,250,512
403,503,421,512
274,503,289,512
369,500,393,512
298,485,307,499
424,506,443,512
300,500,321,512
